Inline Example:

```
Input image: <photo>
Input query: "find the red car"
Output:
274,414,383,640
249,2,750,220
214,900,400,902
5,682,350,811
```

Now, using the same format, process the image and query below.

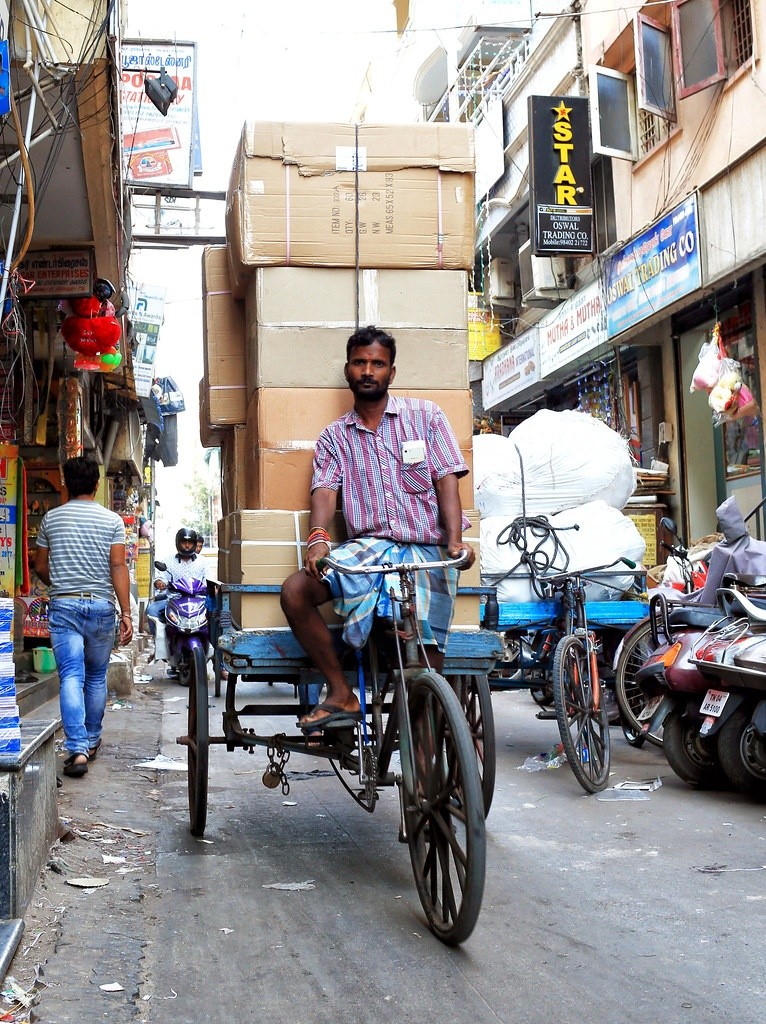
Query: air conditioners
484,257,514,306
519,239,567,301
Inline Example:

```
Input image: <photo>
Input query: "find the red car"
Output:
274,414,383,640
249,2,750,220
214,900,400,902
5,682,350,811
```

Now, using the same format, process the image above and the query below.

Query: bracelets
307,527,331,551
121,609,131,614
119,614,133,623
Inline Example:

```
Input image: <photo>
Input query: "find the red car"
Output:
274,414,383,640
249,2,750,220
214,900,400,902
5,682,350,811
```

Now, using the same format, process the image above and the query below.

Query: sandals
63,755,88,778
88,736,102,760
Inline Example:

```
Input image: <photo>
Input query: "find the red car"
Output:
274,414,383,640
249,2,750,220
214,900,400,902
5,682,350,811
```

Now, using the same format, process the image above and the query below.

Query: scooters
151,561,213,686
613,515,766,797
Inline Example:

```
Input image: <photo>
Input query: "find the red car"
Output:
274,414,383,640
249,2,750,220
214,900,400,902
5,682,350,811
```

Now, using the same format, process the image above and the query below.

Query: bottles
542,744,559,762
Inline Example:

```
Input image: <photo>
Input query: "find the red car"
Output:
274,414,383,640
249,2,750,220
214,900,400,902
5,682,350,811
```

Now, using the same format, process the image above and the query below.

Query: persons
146,528,229,681
279,324,476,840
34,456,133,776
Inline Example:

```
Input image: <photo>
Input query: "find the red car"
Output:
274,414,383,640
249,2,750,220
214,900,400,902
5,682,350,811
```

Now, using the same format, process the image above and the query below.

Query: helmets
176,528,197,556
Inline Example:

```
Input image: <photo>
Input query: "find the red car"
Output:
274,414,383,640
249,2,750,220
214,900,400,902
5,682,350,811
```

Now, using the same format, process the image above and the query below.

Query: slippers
296,702,364,731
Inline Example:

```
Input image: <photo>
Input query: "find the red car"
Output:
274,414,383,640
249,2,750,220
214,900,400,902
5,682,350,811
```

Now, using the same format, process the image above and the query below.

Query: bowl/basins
33,649,57,674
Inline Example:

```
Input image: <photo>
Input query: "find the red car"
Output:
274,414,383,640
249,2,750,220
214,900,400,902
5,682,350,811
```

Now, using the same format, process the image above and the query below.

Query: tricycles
177,550,510,945
479,555,655,793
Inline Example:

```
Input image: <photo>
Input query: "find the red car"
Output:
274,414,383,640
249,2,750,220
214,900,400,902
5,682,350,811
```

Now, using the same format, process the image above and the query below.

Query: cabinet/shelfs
26,462,68,563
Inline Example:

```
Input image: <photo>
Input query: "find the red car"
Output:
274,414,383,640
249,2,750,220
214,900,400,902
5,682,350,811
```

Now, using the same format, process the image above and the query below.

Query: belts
56,593,99,599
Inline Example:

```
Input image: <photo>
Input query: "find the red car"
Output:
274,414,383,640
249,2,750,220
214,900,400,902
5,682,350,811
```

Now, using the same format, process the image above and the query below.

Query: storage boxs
199,118,481,631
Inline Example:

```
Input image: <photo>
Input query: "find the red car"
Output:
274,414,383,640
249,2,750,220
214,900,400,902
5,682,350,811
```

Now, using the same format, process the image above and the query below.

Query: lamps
122,66,178,116
